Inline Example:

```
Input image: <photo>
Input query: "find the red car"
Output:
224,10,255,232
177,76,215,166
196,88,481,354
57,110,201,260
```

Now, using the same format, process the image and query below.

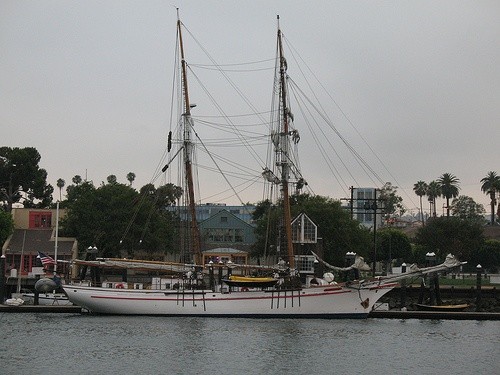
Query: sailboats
13,201,75,305
64,16,467,318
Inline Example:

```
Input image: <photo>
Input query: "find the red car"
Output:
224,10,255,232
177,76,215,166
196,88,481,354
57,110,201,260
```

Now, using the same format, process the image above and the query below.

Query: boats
414,302,471,313
220,276,279,288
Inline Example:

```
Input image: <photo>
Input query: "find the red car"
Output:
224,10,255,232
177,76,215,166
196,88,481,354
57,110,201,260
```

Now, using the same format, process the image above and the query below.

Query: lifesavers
241,287,248,292
116,283,124,288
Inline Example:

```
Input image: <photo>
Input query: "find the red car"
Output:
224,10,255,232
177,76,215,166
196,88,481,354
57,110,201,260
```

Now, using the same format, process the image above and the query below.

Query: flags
38,251,55,264
